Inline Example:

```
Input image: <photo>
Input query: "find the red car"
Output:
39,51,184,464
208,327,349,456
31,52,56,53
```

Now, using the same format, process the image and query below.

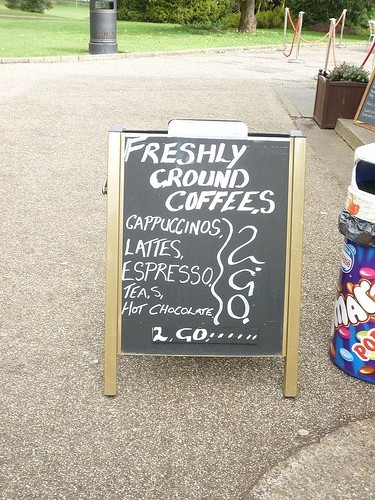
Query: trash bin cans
89,0,117,54
328,142,375,385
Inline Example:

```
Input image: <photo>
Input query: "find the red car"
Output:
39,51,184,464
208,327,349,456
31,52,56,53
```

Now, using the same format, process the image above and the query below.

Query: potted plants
313,61,370,130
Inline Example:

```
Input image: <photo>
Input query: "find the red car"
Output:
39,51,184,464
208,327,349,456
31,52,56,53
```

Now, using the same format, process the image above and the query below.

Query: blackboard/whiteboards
353,66,375,133
104,118,306,358
